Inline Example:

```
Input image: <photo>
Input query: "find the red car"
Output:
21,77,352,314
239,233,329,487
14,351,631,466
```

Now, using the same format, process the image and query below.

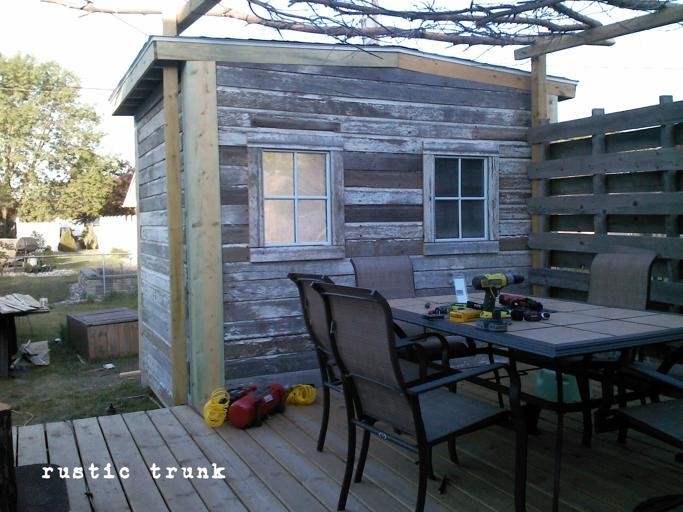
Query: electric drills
472,272,543,318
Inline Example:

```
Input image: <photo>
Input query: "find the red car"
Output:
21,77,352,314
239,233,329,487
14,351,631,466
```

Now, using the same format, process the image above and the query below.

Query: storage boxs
67,307,138,361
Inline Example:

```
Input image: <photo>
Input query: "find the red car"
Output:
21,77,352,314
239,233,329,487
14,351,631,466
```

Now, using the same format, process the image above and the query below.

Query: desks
372,292,683,512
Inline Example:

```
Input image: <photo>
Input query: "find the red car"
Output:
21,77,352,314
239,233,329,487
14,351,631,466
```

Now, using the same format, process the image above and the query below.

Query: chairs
570,251,663,444
309,281,526,511
287,273,456,482
609,345,683,451
350,254,504,411
